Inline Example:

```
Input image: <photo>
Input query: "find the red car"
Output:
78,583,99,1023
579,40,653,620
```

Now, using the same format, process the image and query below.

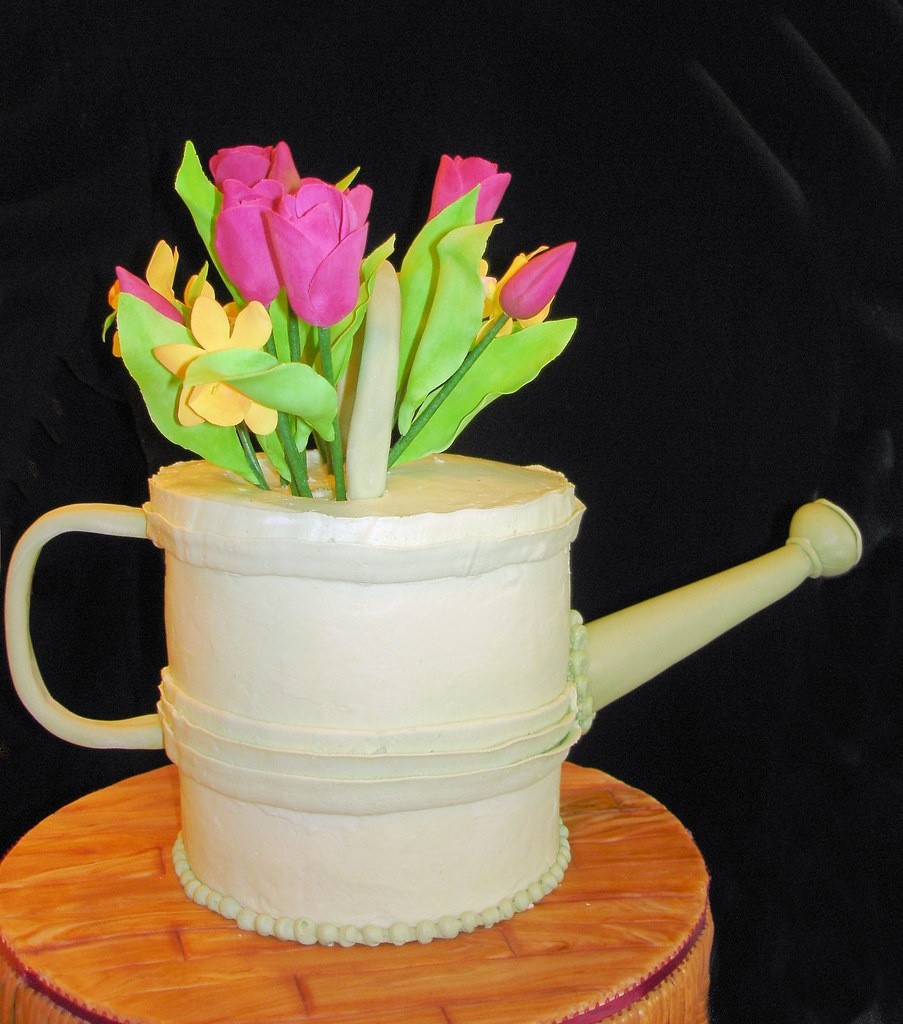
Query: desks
0,760,715,1024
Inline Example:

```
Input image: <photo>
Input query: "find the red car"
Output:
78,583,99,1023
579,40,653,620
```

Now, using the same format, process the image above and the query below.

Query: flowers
82,117,588,504
